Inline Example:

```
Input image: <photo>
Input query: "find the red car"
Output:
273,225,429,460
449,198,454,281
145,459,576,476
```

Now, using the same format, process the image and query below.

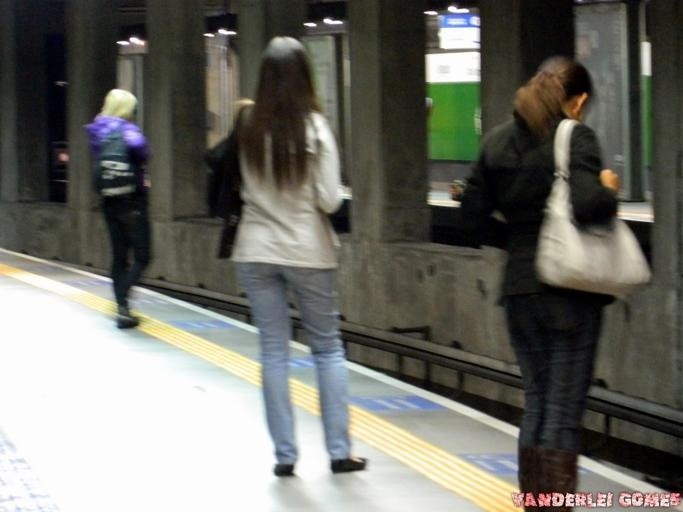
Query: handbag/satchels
534,181,658,299
202,135,245,220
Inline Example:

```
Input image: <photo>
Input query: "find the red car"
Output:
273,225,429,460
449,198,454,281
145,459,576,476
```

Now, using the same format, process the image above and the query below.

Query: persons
83,88,152,329
461,56,621,511
226,38,367,477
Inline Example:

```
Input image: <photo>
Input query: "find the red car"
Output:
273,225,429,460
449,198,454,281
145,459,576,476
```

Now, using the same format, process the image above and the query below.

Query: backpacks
95,124,143,206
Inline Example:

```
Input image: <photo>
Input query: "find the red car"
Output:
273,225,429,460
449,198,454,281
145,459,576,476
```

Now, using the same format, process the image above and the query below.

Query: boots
114,304,140,329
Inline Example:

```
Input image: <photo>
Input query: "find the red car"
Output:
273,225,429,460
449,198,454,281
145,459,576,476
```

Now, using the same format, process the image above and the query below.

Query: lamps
113,20,146,50
302,0,347,29
200,12,241,42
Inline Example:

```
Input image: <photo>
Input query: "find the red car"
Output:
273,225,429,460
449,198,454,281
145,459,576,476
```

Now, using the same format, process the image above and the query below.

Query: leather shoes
330,456,371,474
271,461,297,477
